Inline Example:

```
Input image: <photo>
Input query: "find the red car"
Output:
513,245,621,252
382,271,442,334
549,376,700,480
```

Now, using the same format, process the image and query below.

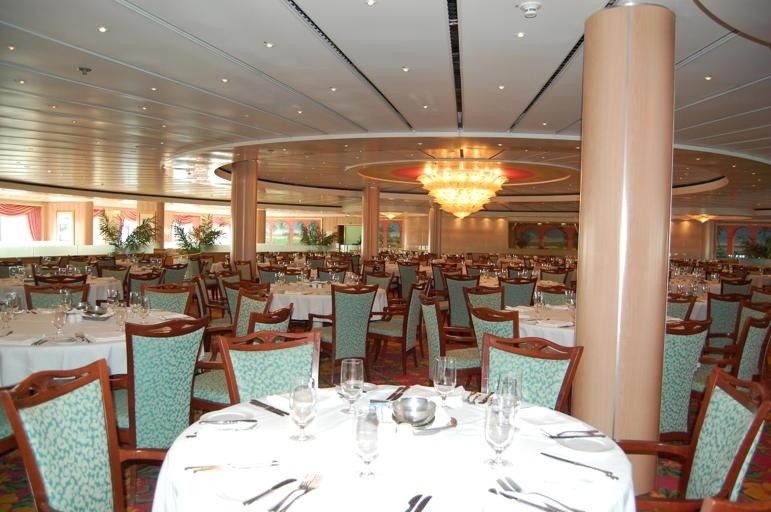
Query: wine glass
289,356,524,485
257,250,336,269
669,257,720,296
1,289,151,337
128,257,162,272
374,248,574,284
275,271,360,288
534,290,576,321
9,264,93,286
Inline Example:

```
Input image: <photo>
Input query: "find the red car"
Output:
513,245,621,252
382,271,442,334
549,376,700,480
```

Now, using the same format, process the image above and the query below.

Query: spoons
543,431,609,439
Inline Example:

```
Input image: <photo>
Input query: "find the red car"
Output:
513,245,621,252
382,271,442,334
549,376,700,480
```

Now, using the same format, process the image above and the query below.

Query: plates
199,409,259,433
555,431,615,451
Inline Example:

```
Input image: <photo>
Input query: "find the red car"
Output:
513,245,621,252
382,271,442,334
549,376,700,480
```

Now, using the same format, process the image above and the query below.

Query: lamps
416,160,510,219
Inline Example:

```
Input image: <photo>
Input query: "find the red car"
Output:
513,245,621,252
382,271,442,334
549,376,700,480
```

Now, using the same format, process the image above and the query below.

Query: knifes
249,385,408,417
198,419,257,424
404,495,433,512
542,453,619,479
243,478,296,507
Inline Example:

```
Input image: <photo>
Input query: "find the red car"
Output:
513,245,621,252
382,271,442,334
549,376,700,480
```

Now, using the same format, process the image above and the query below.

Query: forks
495,478,587,512
540,429,601,433
461,391,480,403
269,472,325,512
